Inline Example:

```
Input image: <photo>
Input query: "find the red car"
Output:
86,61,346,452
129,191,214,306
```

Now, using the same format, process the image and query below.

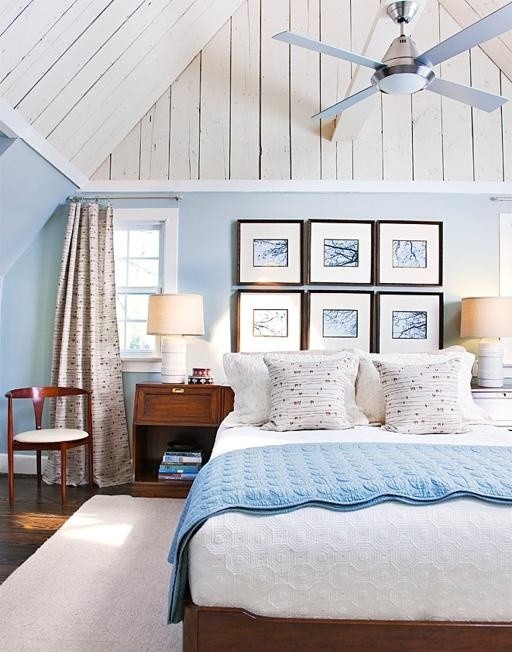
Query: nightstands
130,382,234,498
470,389,512,425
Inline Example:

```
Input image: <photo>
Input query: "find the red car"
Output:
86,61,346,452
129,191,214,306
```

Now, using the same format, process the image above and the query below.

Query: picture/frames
236,218,305,285
305,218,376,285
376,219,444,286
305,290,376,353
376,291,444,354
235,288,305,351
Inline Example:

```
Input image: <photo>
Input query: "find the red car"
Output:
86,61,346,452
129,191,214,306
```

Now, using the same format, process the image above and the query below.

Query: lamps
459,294,511,387
147,294,204,383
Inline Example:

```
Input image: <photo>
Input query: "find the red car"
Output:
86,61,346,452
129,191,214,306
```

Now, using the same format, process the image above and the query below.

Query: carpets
0,493,187,652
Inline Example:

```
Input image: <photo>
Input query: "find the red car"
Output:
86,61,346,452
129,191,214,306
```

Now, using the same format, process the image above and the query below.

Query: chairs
4,386,94,513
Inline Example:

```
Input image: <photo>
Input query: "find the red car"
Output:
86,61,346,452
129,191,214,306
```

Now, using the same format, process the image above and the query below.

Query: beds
163,355,512,652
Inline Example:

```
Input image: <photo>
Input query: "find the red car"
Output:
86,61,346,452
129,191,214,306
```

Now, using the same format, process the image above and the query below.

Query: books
158,450,203,480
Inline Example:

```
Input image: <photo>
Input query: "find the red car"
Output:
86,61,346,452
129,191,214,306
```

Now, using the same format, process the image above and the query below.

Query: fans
272,1,512,121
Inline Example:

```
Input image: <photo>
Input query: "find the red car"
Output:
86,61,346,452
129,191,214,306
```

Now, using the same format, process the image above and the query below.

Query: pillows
224,350,370,429
263,354,360,432
354,345,499,426
371,357,474,434
